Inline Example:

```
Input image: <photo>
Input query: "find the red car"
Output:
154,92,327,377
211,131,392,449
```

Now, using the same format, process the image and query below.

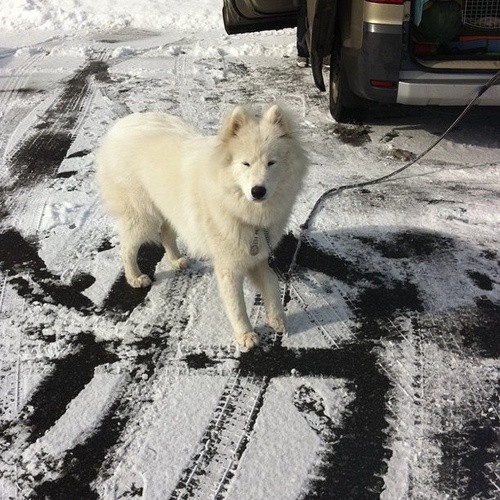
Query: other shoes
297,56,308,68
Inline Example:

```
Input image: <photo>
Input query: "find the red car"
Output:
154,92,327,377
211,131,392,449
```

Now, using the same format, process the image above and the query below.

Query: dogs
95,100,312,349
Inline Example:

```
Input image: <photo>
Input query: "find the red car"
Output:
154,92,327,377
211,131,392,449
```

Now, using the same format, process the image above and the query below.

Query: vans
220,0,499,126
